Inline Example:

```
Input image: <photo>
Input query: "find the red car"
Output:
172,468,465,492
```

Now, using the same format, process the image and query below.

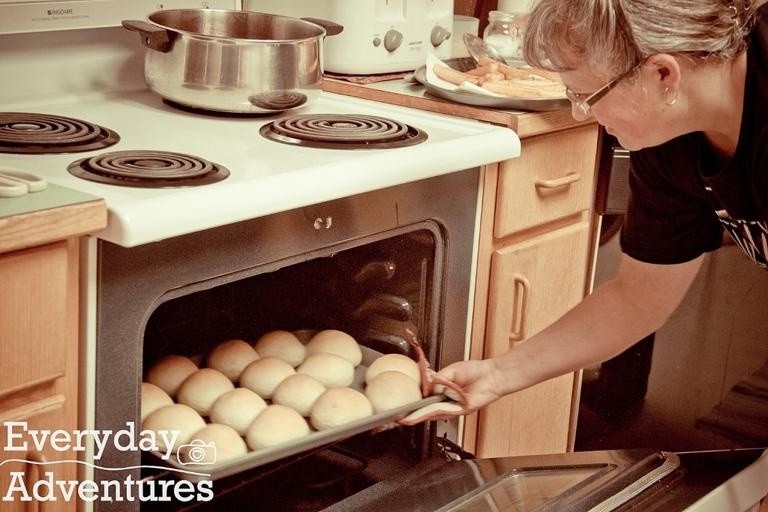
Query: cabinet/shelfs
460,121,602,457
1,235,81,511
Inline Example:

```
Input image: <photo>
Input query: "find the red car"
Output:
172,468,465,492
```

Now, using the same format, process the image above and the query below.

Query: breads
431,53,568,99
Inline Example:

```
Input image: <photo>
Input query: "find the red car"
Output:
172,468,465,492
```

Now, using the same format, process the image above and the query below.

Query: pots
121,7,345,114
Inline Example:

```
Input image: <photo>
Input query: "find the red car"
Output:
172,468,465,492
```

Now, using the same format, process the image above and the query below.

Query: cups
452,15,479,58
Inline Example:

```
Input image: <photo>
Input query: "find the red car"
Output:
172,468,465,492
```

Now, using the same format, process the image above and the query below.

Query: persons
396,0,767,425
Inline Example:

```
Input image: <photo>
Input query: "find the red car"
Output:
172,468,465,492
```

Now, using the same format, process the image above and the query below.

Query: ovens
94,167,768,512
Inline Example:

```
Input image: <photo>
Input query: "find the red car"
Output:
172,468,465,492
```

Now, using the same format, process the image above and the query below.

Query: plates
413,56,571,113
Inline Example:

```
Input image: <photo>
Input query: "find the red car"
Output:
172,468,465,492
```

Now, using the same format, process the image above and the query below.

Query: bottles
483,10,519,60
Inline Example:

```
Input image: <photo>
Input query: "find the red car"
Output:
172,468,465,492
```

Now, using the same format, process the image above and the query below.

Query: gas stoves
0,74,520,249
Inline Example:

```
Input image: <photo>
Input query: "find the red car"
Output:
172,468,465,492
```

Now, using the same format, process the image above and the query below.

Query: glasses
567,58,646,114
